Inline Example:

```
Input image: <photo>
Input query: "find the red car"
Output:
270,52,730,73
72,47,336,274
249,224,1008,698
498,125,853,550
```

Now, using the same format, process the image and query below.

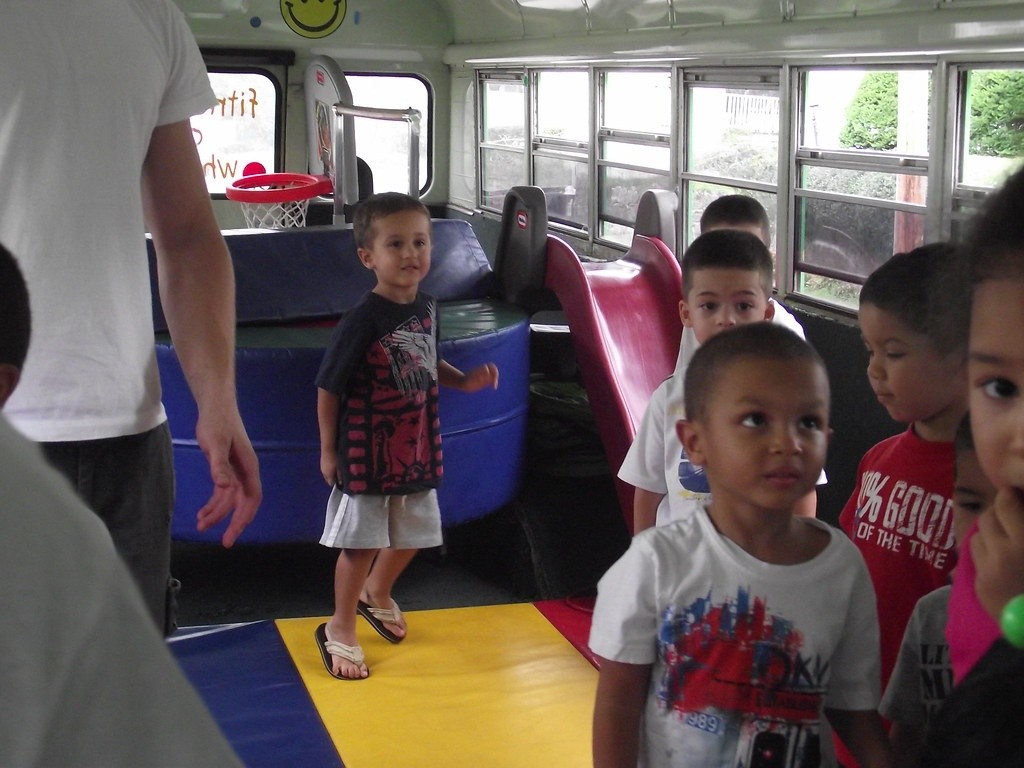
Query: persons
0,0,263,637
877,410,998,768
0,243,239,768
832,243,973,768
312,190,498,681
617,229,828,537
588,320,896,768
674,196,807,390
923,163,1024,768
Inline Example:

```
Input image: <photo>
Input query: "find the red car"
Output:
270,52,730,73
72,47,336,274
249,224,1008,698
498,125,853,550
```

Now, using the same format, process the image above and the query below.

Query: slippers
356,594,407,645
314,623,370,680
